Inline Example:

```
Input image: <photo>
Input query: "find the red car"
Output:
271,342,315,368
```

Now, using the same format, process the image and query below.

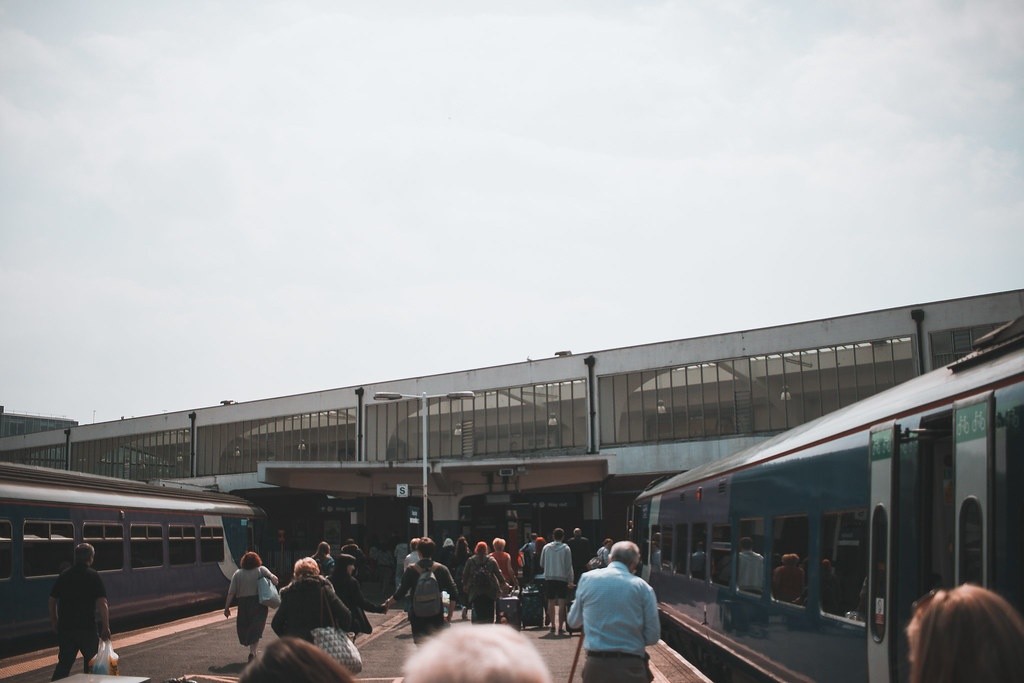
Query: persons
692,532,806,600
907,582,1024,683
51,544,111,682
224,528,661,683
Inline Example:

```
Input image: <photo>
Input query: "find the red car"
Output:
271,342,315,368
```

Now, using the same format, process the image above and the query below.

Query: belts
588,651,640,658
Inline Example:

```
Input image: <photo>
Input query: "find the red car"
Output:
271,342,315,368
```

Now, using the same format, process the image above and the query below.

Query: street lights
374,391,476,538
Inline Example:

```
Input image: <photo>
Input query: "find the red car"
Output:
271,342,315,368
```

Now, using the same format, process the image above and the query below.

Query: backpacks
409,561,443,617
471,555,496,597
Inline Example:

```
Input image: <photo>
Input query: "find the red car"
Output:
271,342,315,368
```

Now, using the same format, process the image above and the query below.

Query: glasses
911,588,936,615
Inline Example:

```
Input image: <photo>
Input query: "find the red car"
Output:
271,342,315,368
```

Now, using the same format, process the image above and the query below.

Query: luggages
499,584,522,631
565,581,585,637
519,577,544,629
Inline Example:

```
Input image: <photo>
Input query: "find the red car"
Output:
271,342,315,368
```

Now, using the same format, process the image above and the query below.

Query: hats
442,537,454,548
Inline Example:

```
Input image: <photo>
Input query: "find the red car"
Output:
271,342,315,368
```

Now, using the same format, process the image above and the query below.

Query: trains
1,463,272,651
632,317,1024,683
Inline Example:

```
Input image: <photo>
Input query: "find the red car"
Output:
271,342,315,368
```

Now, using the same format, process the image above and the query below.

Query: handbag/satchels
584,547,607,573
257,567,281,610
89,638,118,675
310,580,362,676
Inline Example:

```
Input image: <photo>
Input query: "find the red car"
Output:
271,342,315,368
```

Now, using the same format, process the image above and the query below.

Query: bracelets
385,599,392,606
